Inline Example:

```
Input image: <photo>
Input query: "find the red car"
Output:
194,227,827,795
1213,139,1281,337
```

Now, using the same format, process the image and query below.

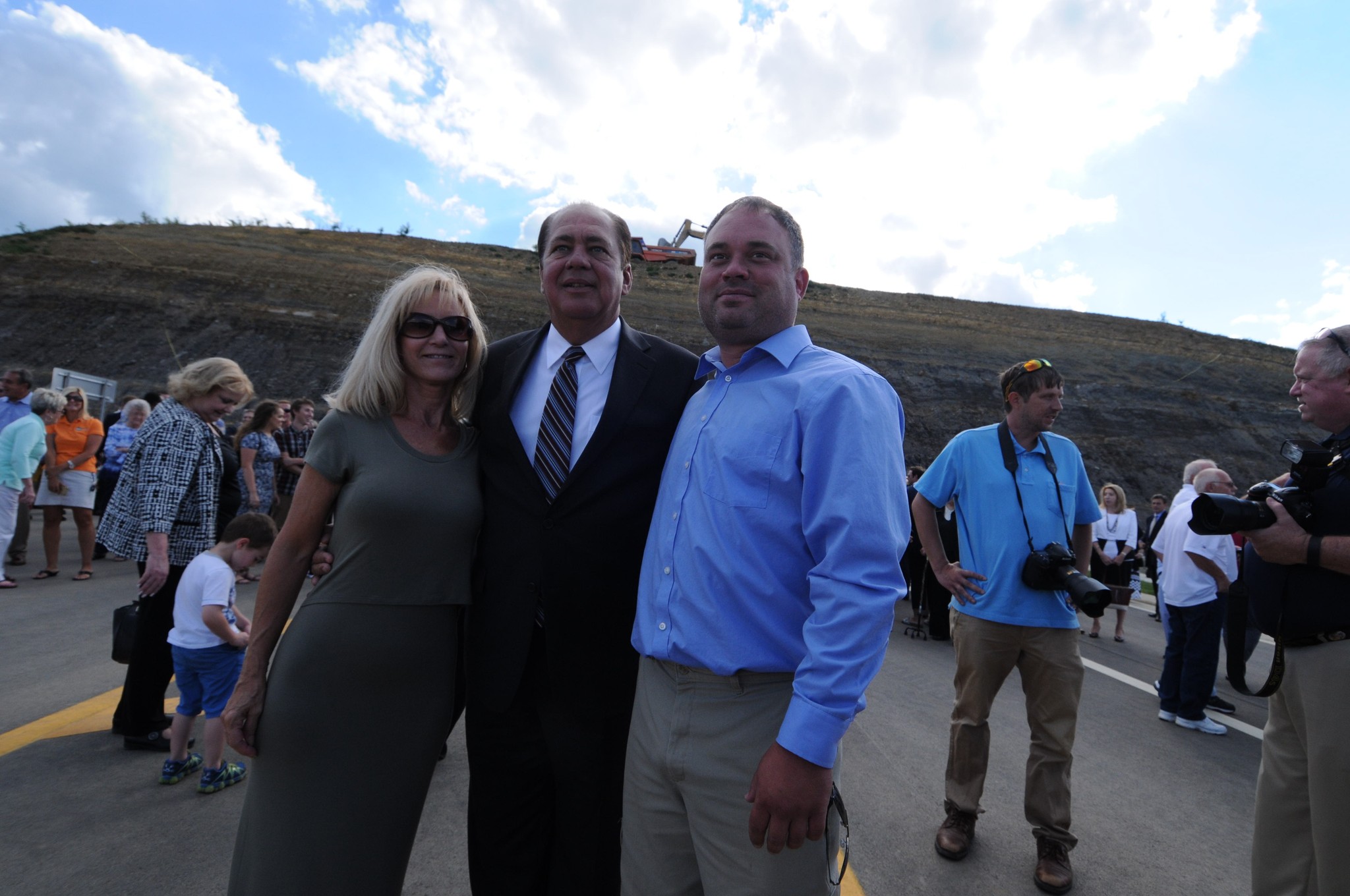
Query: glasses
53,395,83,414
824,780,850,886
1004,358,1052,402
401,311,474,341
1209,479,1235,488
1315,328,1350,358
283,409,291,413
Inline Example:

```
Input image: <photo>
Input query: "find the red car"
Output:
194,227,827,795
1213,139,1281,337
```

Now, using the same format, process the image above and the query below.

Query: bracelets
1306,535,1327,567
1120,551,1127,557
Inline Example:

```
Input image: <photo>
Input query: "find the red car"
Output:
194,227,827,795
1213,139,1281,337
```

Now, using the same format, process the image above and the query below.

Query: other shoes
1079,627,1125,642
91,551,107,559
11,557,25,565
903,613,930,627
1148,612,1162,623
112,554,127,561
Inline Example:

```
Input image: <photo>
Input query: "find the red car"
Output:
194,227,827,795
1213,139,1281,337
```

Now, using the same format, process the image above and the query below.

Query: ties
533,345,585,504
1149,515,1157,536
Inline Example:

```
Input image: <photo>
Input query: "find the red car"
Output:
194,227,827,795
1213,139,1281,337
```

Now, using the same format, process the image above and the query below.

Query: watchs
68,460,75,469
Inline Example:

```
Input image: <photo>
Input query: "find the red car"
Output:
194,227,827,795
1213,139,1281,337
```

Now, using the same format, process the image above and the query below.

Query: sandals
0,574,18,588
236,572,260,584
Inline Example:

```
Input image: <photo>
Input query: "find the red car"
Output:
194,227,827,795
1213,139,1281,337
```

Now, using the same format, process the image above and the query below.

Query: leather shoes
933,813,977,861
1031,838,1074,895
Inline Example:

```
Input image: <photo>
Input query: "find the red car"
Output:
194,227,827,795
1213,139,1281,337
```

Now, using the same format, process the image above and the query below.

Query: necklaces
1106,507,1119,533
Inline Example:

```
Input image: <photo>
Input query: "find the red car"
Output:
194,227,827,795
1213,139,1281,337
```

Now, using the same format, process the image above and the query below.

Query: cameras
1024,541,1113,618
1186,440,1326,536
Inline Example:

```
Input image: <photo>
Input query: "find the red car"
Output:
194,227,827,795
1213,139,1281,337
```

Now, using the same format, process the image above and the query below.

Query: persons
0,358,322,796
221,266,490,896
617,197,913,896
302,202,701,896
899,325,1350,896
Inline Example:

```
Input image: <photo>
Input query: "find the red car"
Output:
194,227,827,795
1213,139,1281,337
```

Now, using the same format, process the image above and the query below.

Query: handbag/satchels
1101,582,1135,611
111,600,141,664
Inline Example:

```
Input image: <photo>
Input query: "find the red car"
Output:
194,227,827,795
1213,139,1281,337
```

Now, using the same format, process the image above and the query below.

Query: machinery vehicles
630,219,708,262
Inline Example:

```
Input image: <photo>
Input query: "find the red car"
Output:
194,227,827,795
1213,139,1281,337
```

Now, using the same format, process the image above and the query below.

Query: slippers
73,570,94,580
33,569,59,579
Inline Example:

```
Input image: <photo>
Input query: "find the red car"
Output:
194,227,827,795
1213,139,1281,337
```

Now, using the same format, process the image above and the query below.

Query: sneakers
1154,680,1235,735
126,714,248,792
